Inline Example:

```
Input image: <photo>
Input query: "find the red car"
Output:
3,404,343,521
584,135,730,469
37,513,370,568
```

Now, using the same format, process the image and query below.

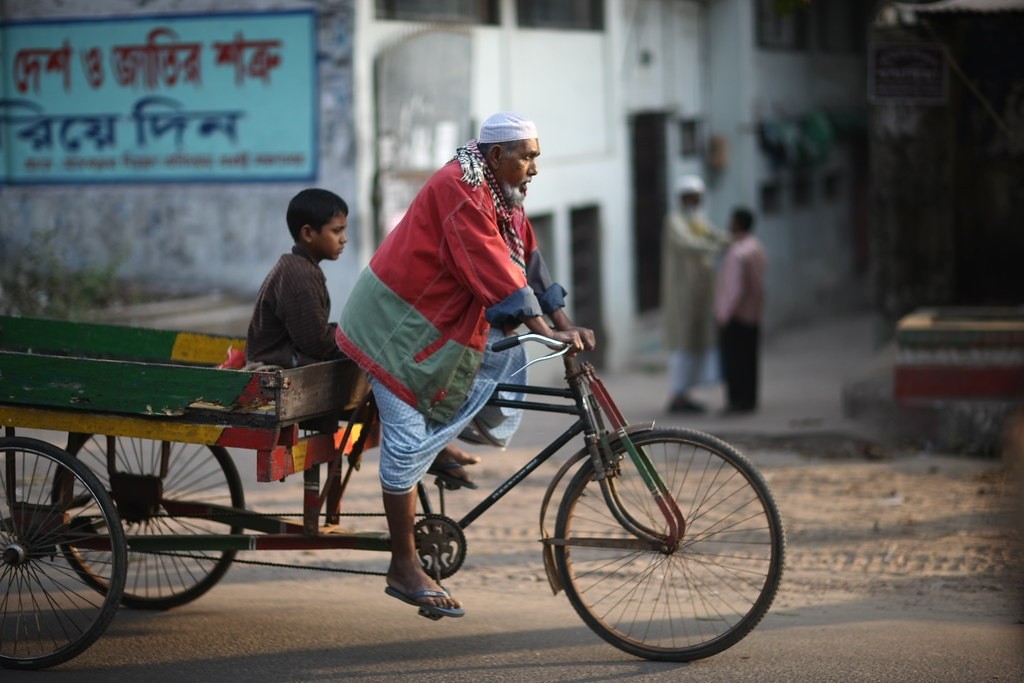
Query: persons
247,189,483,490
662,176,731,413
714,204,767,416
336,113,596,618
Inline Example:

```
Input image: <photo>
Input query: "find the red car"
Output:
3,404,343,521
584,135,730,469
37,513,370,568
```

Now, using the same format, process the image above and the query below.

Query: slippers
384,585,466,617
427,462,479,489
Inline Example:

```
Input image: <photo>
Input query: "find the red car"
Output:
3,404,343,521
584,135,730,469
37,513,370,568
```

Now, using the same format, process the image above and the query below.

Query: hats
478,111,539,143
674,174,704,196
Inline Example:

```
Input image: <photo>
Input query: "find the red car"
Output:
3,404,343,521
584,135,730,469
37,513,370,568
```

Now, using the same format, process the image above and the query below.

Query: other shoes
666,400,707,414
722,405,759,415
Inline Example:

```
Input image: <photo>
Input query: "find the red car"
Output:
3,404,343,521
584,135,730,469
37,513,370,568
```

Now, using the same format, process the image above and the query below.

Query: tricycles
0,312,792,672
